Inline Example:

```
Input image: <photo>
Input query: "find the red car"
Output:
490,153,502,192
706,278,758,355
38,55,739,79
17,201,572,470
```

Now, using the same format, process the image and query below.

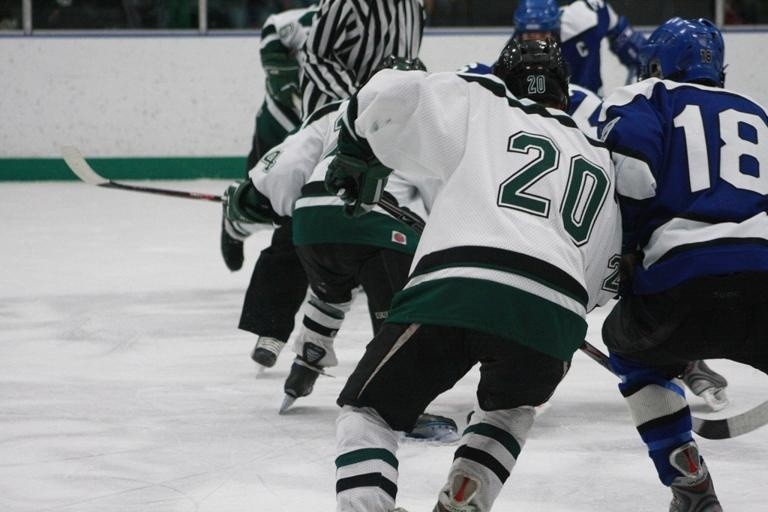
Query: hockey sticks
61,145,228,203
346,179,768,438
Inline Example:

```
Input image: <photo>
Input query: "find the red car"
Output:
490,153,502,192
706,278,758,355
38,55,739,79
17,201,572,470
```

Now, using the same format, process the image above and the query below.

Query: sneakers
684,360,727,394
413,413,456,437
671,472,723,512
252,336,325,397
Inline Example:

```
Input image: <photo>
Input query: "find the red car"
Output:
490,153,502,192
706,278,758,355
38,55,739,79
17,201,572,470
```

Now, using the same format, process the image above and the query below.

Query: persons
225,54,456,446
324,36,623,512
512,1,647,96
459,34,730,411
242,0,427,171
595,15,768,512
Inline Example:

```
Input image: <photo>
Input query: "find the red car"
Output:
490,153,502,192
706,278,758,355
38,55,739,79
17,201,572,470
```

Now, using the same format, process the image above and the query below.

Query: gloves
224,182,273,240
325,122,393,218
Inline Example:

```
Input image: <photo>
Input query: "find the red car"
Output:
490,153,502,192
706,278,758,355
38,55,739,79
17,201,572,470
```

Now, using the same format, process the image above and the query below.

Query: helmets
639,16,725,87
495,38,571,105
375,55,426,71
513,0,563,30
